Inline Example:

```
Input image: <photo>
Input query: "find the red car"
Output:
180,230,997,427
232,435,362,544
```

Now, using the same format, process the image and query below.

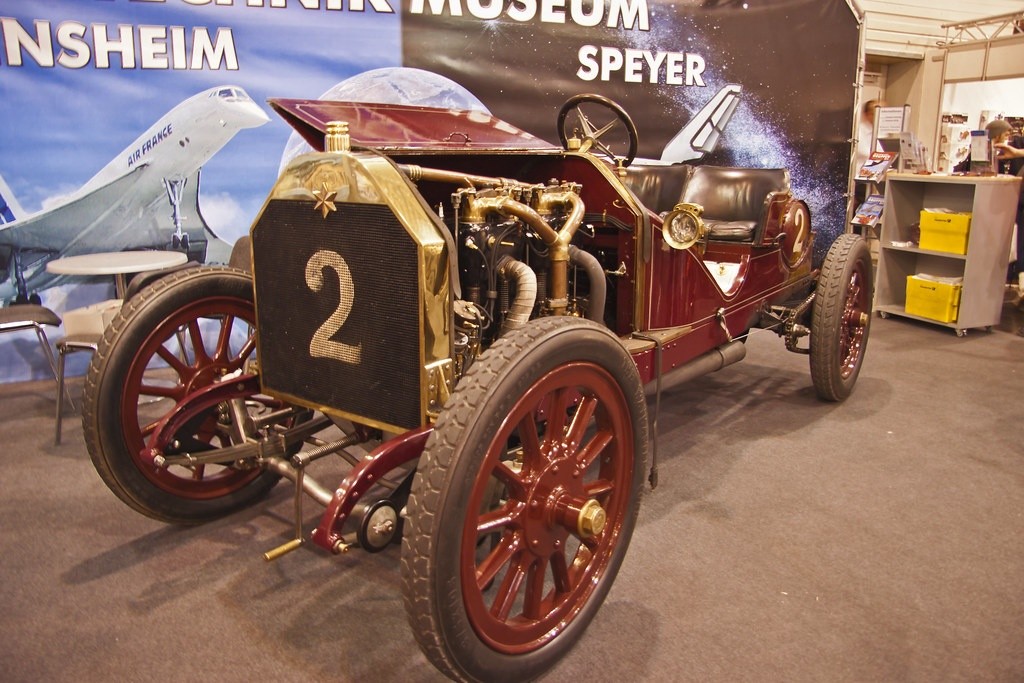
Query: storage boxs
904,275,962,323
918,210,973,255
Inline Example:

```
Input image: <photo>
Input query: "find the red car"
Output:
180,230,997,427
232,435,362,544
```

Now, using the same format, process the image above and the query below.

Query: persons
963,120,1024,271
853,101,884,235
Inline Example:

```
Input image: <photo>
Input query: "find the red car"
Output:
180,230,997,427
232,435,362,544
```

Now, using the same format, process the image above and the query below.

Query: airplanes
0,85,272,307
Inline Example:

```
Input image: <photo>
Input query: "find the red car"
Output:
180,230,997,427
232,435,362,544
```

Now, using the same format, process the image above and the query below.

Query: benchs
617,163,790,299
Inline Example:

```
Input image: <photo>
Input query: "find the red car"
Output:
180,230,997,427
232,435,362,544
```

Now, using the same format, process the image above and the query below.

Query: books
851,150,899,227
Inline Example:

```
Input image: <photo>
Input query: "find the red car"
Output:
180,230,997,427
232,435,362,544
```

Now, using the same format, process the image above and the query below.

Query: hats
986,120,1013,139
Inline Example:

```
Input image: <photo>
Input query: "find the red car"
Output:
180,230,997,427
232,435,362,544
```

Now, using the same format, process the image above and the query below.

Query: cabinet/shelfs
871,172,1023,336
937,112,971,174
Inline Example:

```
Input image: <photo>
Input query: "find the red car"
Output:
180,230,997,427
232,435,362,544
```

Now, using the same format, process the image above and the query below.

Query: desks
44,250,189,300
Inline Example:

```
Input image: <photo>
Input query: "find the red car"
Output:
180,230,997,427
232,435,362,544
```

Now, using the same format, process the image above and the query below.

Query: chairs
54,260,201,448
176,235,252,387
0,304,79,417
